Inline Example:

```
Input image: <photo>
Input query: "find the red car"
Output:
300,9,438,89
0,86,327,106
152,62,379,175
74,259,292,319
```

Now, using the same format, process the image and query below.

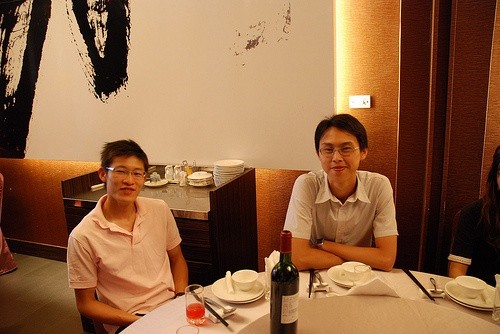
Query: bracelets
315,237,324,249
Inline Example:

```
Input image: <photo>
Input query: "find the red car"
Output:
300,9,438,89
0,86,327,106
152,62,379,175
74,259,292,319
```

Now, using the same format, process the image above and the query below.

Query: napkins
325,278,401,297
264,250,280,293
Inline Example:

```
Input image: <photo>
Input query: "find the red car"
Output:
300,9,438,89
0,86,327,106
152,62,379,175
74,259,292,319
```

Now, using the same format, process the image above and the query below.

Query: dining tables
116,267,500,334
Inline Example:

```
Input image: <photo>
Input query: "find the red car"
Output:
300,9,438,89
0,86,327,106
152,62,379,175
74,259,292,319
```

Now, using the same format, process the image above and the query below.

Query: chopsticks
190,289,229,327
308,268,314,299
402,267,436,302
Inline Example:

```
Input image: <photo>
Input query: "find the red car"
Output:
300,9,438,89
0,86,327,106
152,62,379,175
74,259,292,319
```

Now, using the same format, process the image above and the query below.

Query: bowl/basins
456,275,487,299
341,261,369,282
232,270,259,291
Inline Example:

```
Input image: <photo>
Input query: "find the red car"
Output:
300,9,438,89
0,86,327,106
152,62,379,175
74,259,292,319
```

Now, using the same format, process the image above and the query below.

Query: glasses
106,167,147,180
318,146,360,157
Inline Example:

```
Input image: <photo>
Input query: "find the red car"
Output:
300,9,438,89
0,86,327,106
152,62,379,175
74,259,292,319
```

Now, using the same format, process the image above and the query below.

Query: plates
327,265,377,288
211,278,266,304
444,280,500,311
144,180,168,187
187,159,245,188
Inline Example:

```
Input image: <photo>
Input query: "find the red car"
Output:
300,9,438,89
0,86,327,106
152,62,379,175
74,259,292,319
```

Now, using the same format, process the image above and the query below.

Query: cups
185,284,205,325
149,174,158,183
353,265,371,287
175,325,200,334
492,288,500,323
265,265,272,300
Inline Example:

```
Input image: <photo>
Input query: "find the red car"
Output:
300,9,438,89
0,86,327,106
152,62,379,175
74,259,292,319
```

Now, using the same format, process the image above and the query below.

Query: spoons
201,296,236,313
314,272,328,288
429,277,444,294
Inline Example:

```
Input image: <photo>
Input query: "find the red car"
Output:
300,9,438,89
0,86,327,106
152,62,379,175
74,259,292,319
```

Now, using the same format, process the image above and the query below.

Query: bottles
269,230,299,334
164,164,189,187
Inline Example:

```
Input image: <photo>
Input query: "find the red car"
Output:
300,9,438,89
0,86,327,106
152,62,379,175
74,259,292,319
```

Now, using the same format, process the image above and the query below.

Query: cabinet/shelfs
61,165,258,293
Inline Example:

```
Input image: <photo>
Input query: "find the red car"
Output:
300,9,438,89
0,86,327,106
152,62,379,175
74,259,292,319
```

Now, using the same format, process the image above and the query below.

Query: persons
285,112,398,273
65,139,188,334
447,145,500,286
